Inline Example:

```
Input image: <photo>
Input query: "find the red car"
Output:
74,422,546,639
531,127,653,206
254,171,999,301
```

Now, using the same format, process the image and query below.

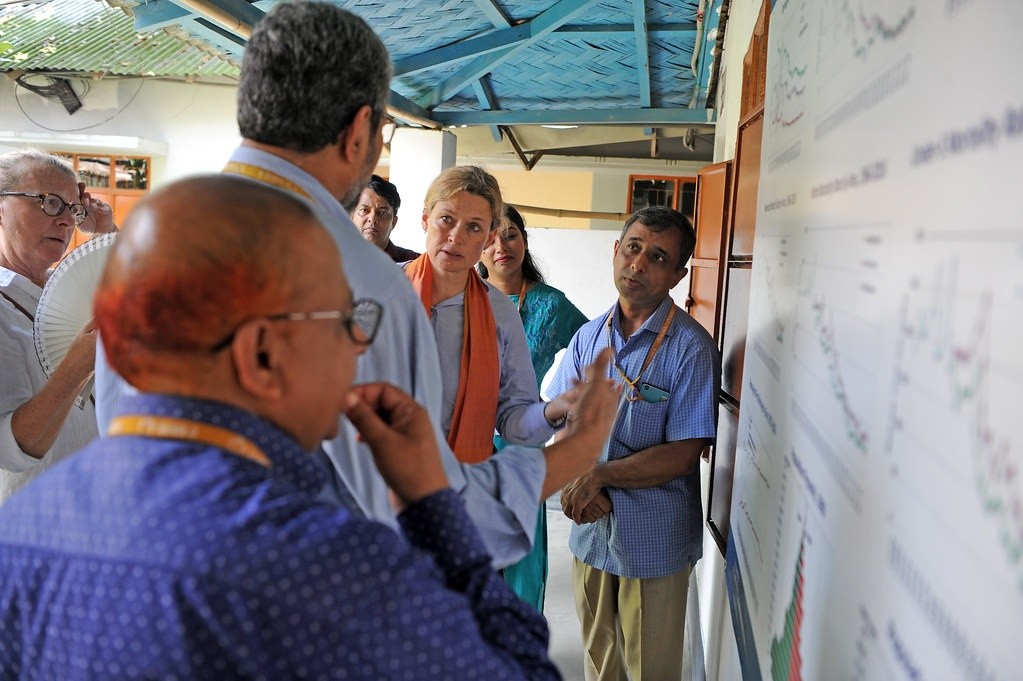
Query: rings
96,199,102,205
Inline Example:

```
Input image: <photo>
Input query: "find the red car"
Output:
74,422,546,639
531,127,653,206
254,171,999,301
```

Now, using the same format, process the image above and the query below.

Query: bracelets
113,223,117,232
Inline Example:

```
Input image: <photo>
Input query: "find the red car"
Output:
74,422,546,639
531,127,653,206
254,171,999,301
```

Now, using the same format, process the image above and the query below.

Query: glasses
331,109,401,146
207,297,383,359
0,190,89,225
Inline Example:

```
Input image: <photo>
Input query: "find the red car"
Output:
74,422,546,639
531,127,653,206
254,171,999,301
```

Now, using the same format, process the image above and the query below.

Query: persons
0,150,120,505
396,166,586,465
0,174,564,681
350,174,421,263
544,206,716,681
478,203,590,614
95,0,622,571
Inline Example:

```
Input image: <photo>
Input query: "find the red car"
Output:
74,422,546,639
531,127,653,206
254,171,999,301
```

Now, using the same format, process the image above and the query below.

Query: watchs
547,416,564,427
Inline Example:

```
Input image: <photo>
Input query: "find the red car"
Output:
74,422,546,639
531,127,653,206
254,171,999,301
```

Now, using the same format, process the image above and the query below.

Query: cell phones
639,383,671,403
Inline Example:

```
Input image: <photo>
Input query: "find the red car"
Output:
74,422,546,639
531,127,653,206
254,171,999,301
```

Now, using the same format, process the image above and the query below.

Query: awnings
106,0,721,142
